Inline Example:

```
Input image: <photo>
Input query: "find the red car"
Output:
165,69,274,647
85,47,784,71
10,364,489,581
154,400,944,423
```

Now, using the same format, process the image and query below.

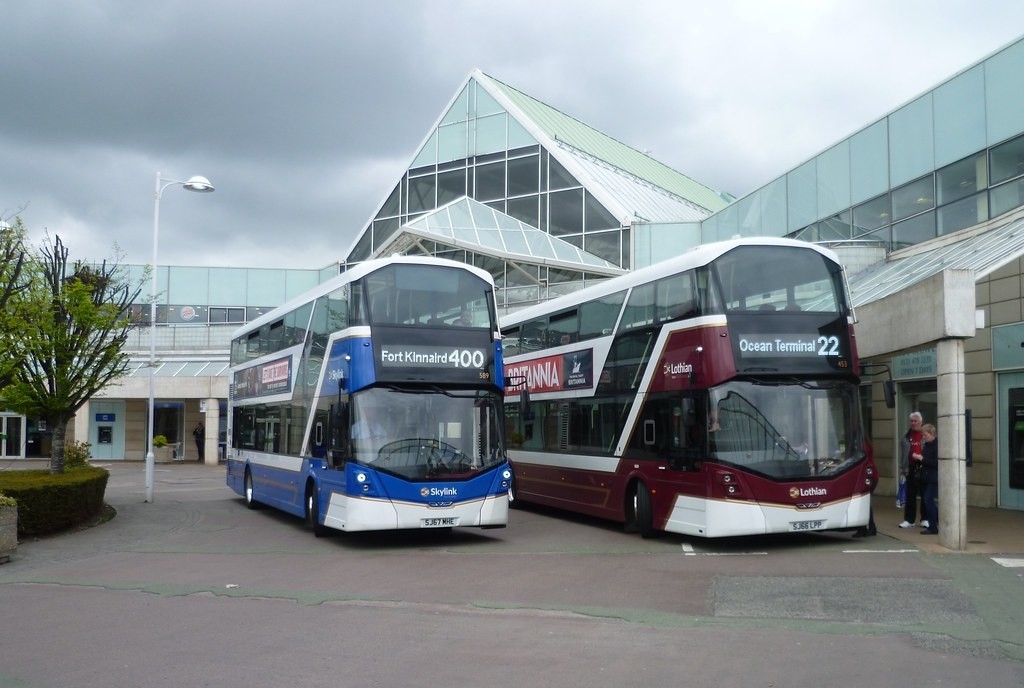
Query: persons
709,402,722,433
351,407,386,440
193,423,204,460
898,411,929,528
452,310,472,327
912,423,938,534
851,438,877,538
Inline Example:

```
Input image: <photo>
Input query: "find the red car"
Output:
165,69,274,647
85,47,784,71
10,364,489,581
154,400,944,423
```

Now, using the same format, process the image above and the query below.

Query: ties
369,427,375,454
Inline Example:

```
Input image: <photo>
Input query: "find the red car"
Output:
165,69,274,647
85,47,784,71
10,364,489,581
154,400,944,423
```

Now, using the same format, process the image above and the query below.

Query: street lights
143,170,218,503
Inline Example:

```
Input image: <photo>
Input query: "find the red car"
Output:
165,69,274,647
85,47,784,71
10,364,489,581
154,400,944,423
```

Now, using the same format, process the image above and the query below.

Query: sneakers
899,520,916,528
920,519,929,527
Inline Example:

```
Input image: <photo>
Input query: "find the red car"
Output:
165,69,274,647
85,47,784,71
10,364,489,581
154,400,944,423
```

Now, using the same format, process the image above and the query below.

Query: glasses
461,314,469,317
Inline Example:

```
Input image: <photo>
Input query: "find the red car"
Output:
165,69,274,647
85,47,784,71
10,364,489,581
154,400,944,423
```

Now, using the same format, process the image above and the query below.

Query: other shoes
920,526,938,534
852,530,876,537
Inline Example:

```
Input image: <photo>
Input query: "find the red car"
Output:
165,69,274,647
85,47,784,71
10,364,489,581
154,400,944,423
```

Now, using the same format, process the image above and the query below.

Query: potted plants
0,492,20,566
152,436,173,465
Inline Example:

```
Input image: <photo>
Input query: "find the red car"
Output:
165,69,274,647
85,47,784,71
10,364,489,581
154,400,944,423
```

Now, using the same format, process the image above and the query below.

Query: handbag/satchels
912,457,932,484
895,474,907,509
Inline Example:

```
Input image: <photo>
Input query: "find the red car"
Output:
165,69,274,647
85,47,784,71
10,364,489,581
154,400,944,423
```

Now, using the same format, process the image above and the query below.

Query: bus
215,257,531,538
478,236,901,540
